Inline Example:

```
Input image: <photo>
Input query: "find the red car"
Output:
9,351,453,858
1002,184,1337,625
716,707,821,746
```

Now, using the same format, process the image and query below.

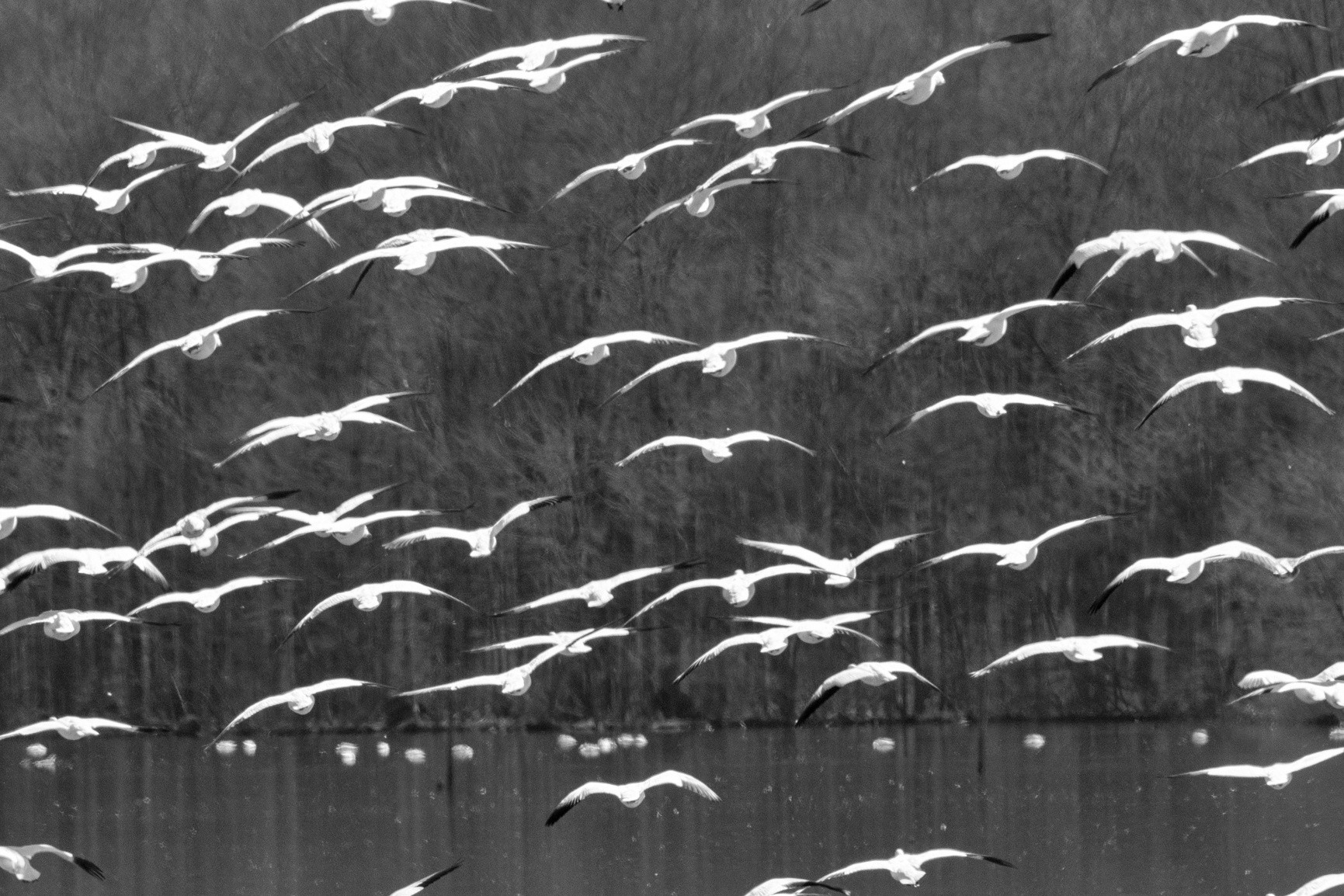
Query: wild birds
0,0,1344,896
